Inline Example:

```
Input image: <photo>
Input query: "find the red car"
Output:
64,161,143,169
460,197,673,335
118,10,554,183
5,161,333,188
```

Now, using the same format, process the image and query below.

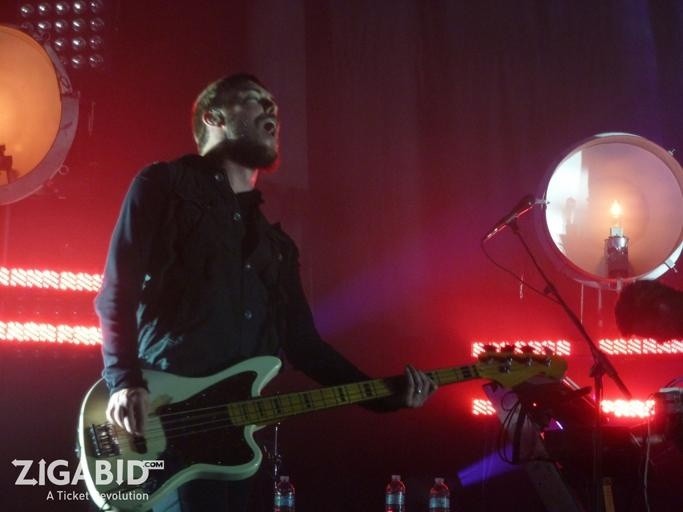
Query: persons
94,71,440,511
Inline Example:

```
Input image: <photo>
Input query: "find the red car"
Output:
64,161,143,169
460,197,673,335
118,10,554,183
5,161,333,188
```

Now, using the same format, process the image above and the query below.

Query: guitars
76,344,567,512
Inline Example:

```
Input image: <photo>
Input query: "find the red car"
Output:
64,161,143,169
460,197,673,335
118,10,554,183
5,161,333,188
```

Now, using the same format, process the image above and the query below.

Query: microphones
483,193,536,243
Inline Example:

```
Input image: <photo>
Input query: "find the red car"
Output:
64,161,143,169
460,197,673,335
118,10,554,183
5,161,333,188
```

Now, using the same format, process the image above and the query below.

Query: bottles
603,226,629,278
273,475,298,512
0,143,14,187
385,474,406,512
427,477,451,512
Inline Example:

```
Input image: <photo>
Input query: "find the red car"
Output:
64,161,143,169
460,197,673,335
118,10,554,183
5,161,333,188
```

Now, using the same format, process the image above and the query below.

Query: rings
415,390,422,396
